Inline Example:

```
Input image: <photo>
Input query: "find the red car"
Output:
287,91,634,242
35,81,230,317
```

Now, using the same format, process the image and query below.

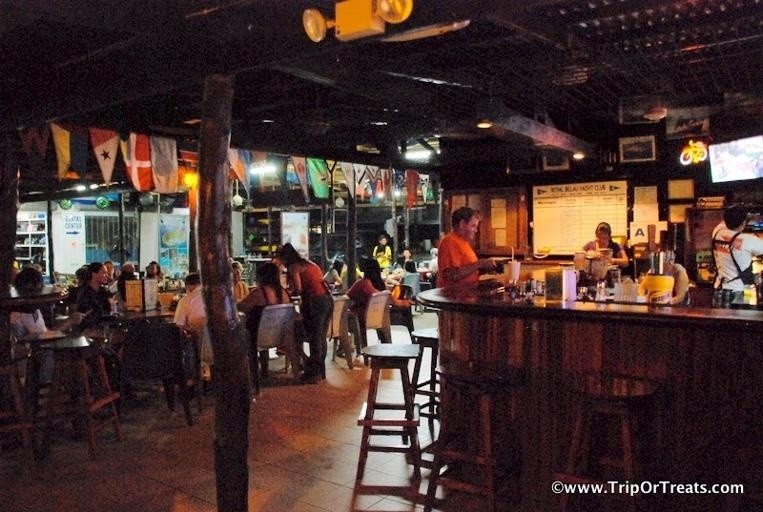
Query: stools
356,328,660,511
0,331,123,460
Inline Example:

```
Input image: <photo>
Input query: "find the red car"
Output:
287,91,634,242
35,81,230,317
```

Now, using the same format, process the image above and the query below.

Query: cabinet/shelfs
14,211,50,276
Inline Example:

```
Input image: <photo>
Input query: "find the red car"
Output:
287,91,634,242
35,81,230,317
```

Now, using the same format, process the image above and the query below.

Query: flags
306,158,330,199
120,131,155,193
150,136,178,194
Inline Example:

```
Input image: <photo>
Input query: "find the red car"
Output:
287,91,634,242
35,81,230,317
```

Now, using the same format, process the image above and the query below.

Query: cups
562,269,577,304
647,273,674,305
158,294,173,311
650,251,663,275
508,262,521,284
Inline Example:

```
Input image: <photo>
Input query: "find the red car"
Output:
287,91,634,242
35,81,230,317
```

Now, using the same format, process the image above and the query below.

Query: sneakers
293,371,327,385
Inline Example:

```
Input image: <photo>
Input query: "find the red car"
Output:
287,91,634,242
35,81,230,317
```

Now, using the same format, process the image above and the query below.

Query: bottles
511,278,546,299
578,249,645,303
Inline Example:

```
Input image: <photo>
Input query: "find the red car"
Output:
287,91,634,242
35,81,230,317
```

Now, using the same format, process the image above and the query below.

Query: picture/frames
619,135,656,163
543,150,570,171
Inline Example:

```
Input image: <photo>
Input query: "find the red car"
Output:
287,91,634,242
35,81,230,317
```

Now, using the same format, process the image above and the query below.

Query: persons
436,207,497,288
581,222,629,268
712,204,763,307
639,250,690,305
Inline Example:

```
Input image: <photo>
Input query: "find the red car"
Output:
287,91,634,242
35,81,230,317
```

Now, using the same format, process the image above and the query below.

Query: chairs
111,272,438,426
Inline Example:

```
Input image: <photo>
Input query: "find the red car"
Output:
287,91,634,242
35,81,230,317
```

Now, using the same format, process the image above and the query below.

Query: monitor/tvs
707,133,763,185
